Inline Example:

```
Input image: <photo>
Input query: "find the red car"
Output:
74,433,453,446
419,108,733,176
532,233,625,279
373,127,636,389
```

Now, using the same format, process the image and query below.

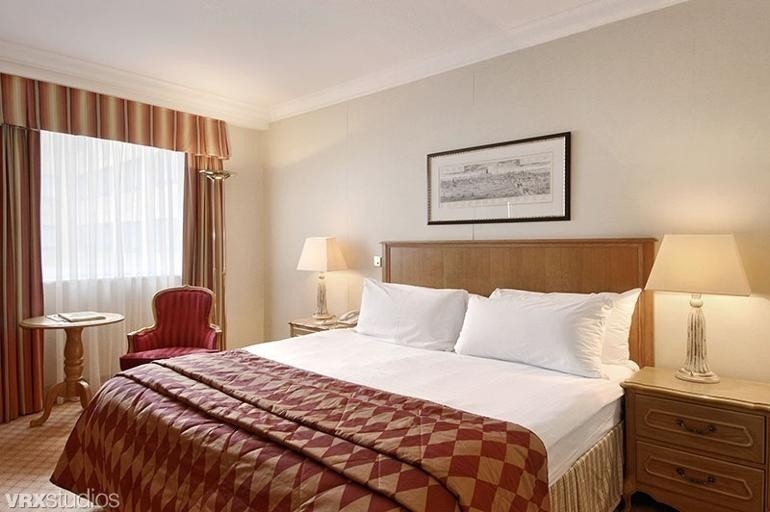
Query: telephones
336,310,360,326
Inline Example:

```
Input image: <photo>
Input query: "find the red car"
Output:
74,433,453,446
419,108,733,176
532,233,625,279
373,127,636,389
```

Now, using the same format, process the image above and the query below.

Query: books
46,311,104,322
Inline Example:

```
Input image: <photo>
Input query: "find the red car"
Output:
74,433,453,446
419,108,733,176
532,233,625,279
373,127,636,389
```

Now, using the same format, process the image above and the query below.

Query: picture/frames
422,131,572,224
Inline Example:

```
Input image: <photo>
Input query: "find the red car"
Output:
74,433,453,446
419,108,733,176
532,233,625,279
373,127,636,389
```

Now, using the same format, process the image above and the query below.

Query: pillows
354,270,646,391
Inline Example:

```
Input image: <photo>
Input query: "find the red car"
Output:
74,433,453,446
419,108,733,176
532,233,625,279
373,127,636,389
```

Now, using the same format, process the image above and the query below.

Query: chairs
119,284,223,368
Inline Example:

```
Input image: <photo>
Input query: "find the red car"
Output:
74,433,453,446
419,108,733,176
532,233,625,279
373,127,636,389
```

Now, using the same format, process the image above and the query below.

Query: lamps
639,233,755,384
296,234,352,322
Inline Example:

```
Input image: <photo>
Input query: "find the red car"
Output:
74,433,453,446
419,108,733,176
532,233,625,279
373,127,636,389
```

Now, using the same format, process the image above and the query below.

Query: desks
17,309,128,428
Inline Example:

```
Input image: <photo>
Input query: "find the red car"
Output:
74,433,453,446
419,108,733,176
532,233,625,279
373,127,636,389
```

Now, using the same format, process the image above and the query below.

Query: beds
95,237,659,511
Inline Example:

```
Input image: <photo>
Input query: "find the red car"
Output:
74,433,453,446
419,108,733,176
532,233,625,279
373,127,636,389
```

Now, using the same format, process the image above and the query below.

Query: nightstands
290,312,349,338
619,363,769,511
